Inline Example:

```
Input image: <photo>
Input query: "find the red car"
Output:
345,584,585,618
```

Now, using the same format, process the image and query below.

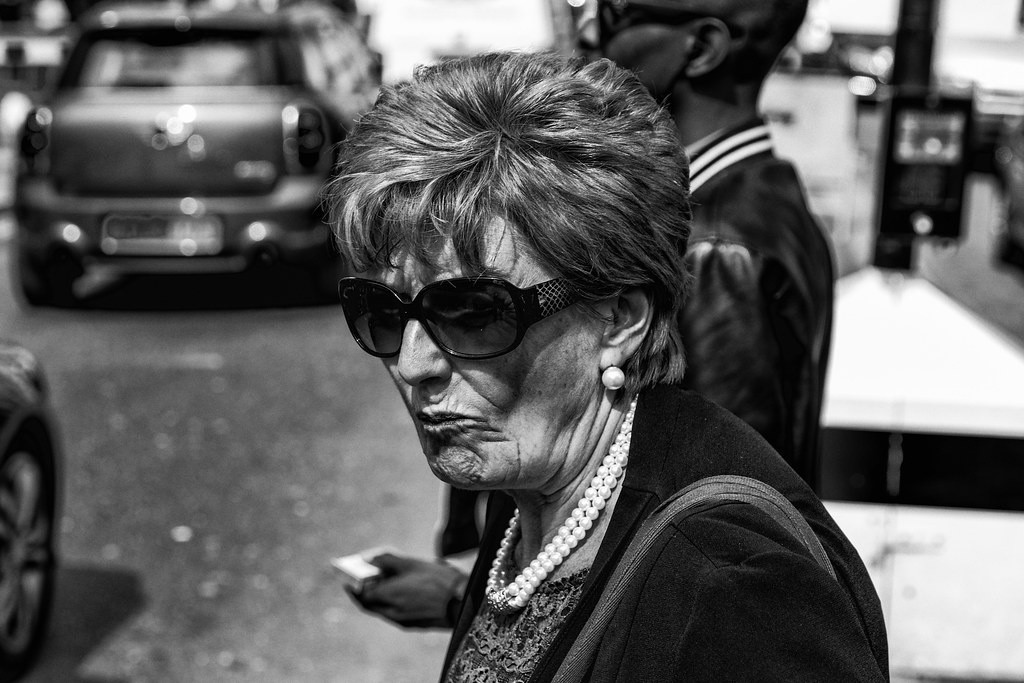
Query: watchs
444,576,470,628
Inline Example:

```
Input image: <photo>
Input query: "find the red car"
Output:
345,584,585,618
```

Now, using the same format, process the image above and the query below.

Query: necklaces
485,392,638,615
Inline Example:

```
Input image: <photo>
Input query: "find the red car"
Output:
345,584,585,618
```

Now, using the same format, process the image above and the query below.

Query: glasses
598,0,747,55
338,268,610,360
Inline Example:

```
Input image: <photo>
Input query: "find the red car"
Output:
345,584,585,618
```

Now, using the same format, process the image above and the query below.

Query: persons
327,52,890,683
343,0,836,631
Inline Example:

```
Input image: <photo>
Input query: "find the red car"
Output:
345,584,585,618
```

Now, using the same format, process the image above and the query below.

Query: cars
6,0,385,309
0,338,62,683
